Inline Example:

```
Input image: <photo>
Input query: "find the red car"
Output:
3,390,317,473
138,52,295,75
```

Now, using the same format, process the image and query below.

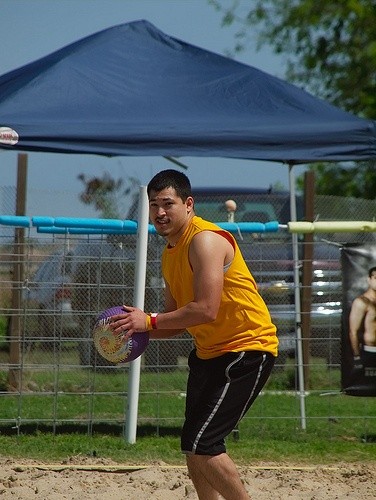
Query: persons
348,266,376,376
106,170,279,500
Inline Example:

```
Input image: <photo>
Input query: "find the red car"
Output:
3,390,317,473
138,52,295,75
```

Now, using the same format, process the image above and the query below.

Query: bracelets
145,313,159,331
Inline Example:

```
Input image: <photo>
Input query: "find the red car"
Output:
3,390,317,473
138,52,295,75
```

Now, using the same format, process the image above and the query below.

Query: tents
0,19,376,429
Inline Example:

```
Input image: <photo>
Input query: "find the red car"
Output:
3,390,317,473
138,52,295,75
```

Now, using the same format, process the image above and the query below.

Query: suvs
74,186,347,375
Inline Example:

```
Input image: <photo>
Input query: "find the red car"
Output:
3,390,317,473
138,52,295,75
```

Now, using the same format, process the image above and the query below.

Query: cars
5,242,148,348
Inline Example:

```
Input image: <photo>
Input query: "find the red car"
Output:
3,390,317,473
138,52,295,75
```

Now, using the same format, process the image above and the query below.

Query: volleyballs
92,306,151,364
